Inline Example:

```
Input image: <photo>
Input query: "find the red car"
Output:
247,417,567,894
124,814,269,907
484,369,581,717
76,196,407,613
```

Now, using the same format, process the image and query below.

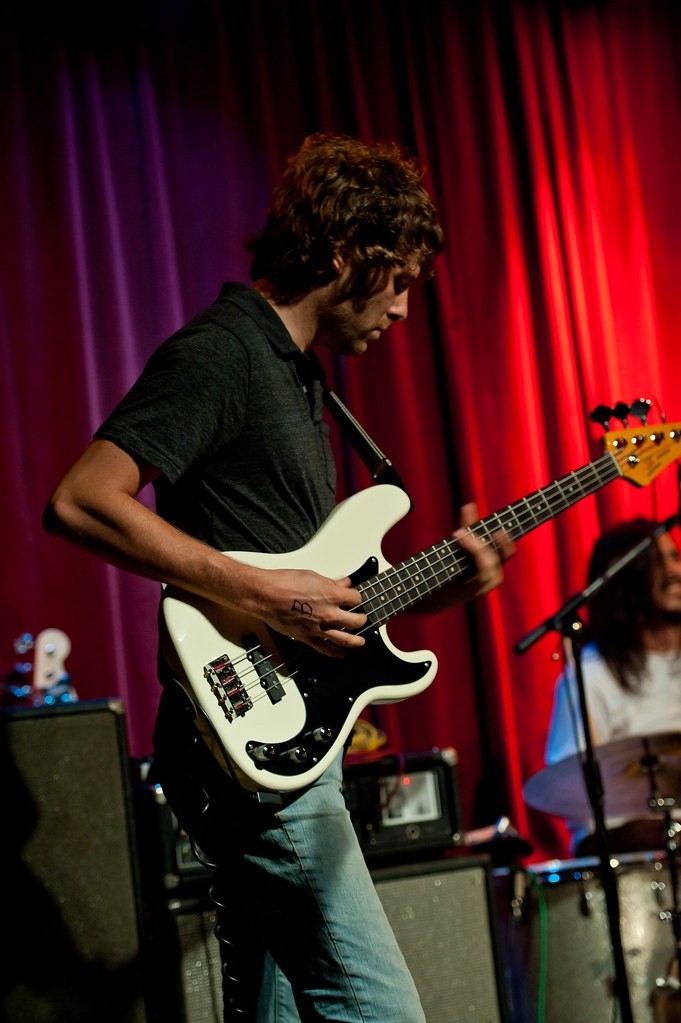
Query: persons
43,138,515,1023
546,517,681,853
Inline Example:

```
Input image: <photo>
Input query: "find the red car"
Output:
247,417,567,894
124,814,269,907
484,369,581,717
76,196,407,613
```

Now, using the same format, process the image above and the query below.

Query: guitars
12,626,77,705
154,397,680,814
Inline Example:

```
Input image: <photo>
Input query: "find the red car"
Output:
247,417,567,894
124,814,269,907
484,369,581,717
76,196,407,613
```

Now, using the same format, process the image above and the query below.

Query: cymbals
522,730,681,816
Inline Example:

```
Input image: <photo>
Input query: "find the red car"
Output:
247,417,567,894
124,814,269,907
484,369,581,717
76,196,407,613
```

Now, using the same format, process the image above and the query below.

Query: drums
520,848,680,1023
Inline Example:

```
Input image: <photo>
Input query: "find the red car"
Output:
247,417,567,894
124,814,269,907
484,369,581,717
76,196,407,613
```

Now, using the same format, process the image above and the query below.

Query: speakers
167,853,514,1023
0,696,159,1023
